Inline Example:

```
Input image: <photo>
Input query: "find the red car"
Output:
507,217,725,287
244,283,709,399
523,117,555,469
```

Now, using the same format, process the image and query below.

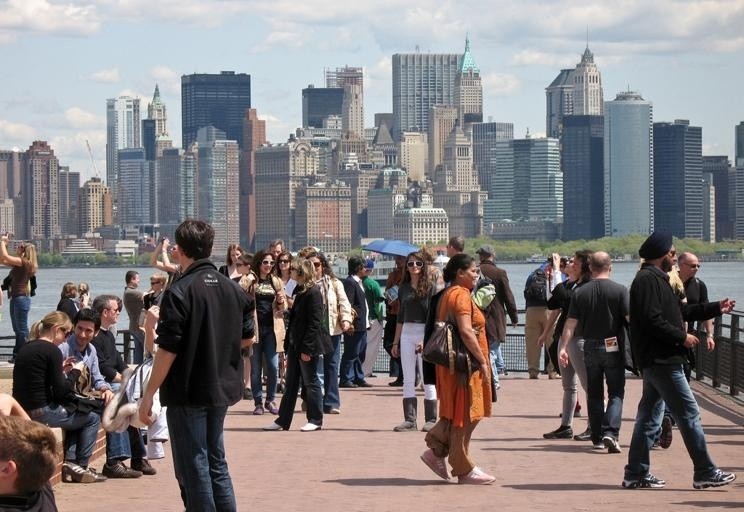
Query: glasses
262,260,275,266
314,262,321,266
408,261,423,267
684,263,701,268
278,260,288,263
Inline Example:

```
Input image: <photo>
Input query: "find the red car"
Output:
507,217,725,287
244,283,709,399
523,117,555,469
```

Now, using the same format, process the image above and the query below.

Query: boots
422,399,437,432
393,397,417,431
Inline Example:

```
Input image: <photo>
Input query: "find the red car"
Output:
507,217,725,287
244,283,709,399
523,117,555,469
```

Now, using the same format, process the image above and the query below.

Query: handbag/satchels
422,320,480,375
337,302,358,328
472,267,496,310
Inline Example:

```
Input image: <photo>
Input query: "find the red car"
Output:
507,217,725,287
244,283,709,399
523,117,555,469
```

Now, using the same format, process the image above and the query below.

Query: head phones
297,266,304,276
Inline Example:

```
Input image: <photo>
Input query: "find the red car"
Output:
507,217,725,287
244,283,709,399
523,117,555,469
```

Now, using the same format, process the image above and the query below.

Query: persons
386,235,519,484
525,231,736,488
220,239,387,431
1,222,256,511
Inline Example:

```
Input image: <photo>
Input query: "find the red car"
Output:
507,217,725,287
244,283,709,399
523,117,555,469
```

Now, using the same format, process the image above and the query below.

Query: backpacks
524,268,547,303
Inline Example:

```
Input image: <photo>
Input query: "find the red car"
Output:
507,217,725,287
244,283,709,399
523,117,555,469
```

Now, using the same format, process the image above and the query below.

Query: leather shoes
264,422,281,430
300,423,321,431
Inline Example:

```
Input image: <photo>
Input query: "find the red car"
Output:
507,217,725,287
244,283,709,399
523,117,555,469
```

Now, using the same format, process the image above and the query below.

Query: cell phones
415,346,421,354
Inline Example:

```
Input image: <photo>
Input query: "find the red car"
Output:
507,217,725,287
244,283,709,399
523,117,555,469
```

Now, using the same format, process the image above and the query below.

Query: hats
476,245,495,257
639,229,672,258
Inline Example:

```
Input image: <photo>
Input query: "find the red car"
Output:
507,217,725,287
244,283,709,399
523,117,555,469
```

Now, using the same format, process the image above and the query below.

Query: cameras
144,294,154,309
160,236,166,244
5,232,13,238
548,256,569,269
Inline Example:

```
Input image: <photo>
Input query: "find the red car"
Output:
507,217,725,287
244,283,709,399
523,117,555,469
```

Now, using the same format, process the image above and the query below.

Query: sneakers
544,426,621,453
62,459,156,483
458,466,496,484
622,473,665,489
330,408,339,414
529,373,561,378
420,449,448,479
340,381,371,387
693,469,735,489
254,401,278,415
660,417,672,448
389,381,404,386
